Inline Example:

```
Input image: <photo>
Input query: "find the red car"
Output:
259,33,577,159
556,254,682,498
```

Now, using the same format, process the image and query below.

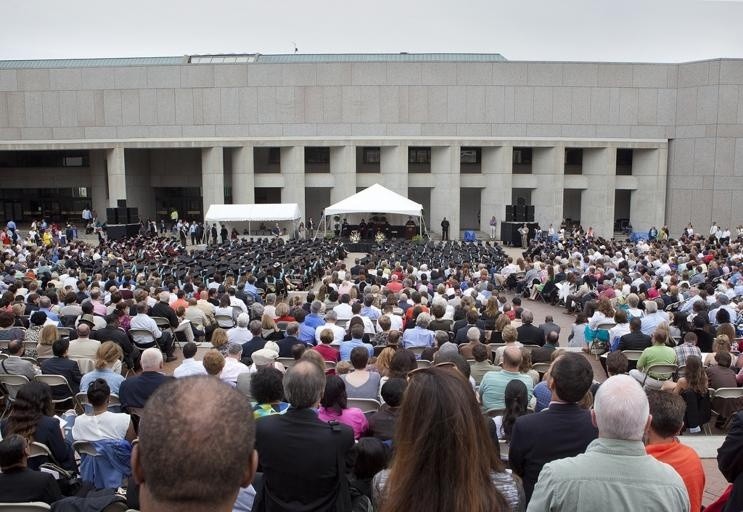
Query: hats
78,314,95,326
251,348,279,366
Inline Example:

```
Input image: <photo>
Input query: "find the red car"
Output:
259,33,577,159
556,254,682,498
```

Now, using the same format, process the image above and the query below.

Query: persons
1,206,742,512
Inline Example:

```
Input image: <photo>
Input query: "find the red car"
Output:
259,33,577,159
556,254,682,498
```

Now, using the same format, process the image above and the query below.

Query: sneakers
563,311,573,314
194,342,201,346
166,356,177,361
582,348,589,353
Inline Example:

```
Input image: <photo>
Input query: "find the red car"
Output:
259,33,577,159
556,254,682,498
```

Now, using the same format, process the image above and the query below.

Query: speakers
106,208,116,224
516,205,526,222
526,205,534,222
128,208,139,224
116,207,128,224
516,196,526,205
117,199,126,208
506,205,515,222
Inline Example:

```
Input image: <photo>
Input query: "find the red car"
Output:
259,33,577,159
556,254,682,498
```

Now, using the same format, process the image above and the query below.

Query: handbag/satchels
38,462,75,479
589,341,610,355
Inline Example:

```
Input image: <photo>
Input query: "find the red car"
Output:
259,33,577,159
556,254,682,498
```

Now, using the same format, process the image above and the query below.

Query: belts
647,373,672,381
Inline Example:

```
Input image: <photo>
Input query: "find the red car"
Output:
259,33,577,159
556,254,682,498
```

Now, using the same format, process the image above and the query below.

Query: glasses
407,361,457,381
